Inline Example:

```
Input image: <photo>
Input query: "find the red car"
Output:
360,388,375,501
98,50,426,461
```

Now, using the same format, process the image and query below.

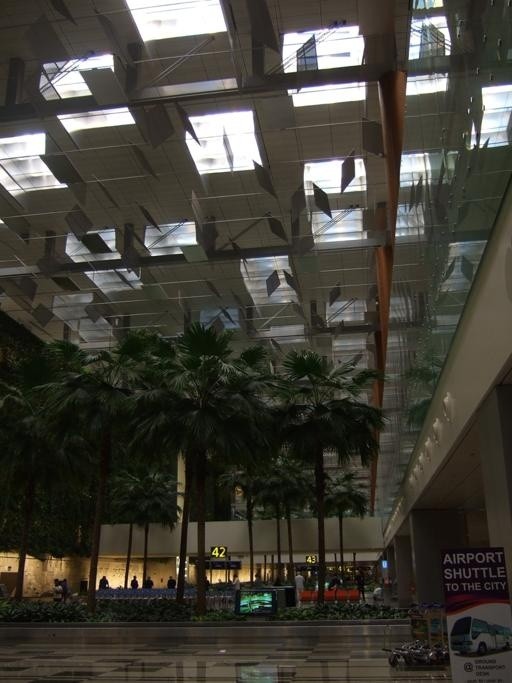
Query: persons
353,569,367,601
51,578,65,601
205,575,211,591
254,573,262,584
231,572,241,591
130,574,140,589
408,586,419,608
60,578,68,593
98,575,110,589
294,570,306,605
144,574,155,589
166,575,177,589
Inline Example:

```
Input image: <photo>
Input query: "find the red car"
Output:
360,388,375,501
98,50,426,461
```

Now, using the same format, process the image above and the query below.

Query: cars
408,601,446,619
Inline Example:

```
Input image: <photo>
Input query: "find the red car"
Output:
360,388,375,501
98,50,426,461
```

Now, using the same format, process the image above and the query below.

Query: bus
450,615,512,655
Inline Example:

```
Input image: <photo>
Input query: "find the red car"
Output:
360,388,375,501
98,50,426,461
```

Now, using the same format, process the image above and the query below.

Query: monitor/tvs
235,590,276,615
